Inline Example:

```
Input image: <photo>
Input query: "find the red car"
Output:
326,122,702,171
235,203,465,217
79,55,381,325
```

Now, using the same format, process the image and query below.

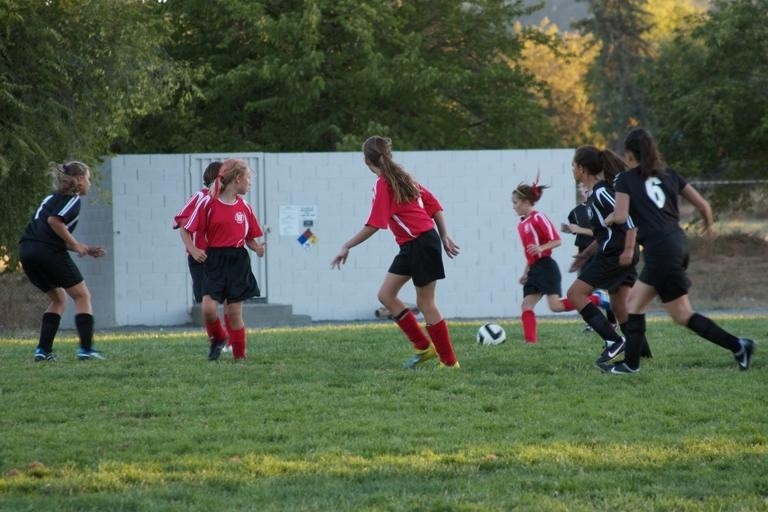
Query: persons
566,145,653,365
330,136,460,368
18,161,105,359
603,129,755,375
172,162,234,351
561,181,615,332
510,172,599,348
180,158,267,364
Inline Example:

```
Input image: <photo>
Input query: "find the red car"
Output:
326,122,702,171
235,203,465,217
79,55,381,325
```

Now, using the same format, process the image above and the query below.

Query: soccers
477,325,506,347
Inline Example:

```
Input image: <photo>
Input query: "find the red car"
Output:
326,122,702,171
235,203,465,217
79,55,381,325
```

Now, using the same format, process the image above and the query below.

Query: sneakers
594,288,611,310
208,339,228,362
76,345,110,361
735,338,754,371
33,348,59,363
595,336,629,365
601,366,639,378
431,361,462,373
403,342,437,370
